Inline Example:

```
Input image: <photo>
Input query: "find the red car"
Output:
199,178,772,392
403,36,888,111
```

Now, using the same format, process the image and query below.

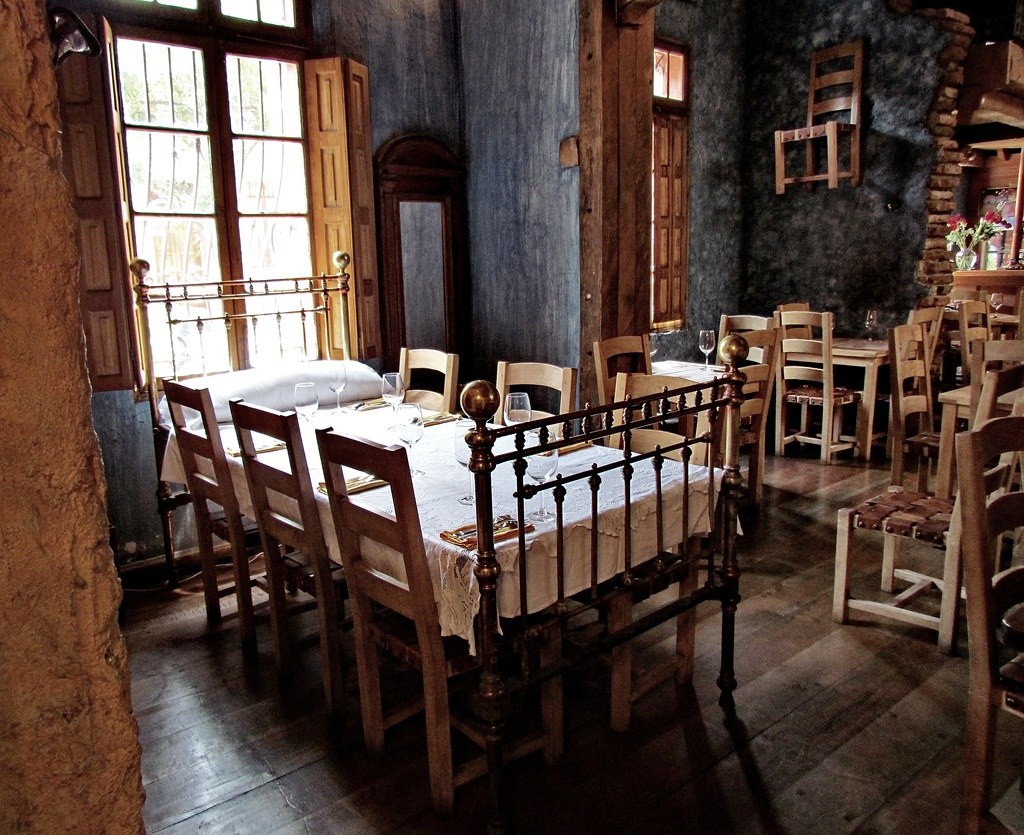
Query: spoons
459,521,518,539
454,514,511,539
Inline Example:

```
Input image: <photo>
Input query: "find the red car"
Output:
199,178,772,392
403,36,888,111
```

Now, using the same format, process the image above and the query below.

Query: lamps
47,5,102,67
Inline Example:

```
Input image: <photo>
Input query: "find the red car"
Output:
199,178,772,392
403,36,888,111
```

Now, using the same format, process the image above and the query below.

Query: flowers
946,210,1012,268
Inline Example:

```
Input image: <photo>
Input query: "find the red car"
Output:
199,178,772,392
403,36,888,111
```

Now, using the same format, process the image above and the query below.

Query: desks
815,338,918,461
651,360,728,383
129,250,750,835
934,386,1023,549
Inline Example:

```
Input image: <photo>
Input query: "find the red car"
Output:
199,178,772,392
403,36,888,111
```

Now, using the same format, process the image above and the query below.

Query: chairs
162,290,1024,835
775,38,865,193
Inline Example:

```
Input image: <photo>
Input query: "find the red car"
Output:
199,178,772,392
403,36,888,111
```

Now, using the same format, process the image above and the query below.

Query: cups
504,392,531,427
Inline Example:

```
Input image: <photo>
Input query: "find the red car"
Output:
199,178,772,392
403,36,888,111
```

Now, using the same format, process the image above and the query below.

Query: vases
955,248,977,272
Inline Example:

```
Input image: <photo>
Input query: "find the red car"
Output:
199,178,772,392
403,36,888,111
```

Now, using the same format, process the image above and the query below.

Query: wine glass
865,310,877,343
397,403,425,477
991,293,1003,318
650,334,658,374
455,421,476,505
526,431,558,523
825,312,838,348
382,373,405,431
328,366,348,415
699,330,715,371
295,383,319,422
